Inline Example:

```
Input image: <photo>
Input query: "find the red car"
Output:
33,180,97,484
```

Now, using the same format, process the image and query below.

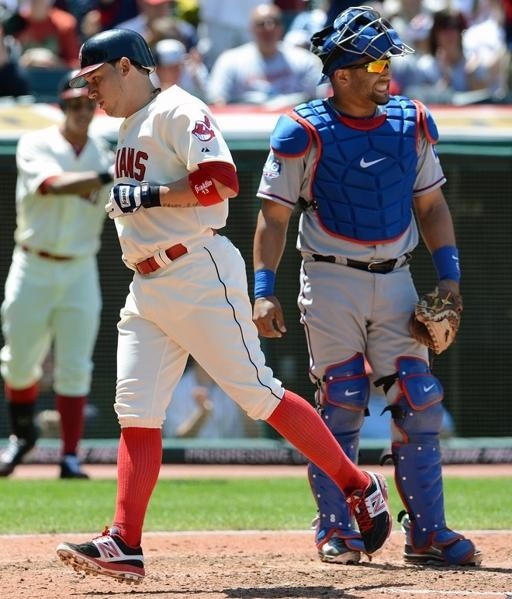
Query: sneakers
344,470,393,556
0,427,43,476
403,517,475,562
311,516,360,565
60,456,90,478
57,527,145,584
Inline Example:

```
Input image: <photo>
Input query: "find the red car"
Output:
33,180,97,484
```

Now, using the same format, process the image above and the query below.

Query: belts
135,244,188,275
312,252,397,274
21,245,73,260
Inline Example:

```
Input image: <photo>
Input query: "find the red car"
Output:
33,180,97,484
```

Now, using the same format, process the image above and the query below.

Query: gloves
105,182,161,219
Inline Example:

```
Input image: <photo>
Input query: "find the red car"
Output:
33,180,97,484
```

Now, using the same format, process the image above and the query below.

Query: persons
0,67,114,482
1,1,512,106
34,342,102,441
54,26,391,585
359,356,456,441
250,1,486,570
162,360,257,441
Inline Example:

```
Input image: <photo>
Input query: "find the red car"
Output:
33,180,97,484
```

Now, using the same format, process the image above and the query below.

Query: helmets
59,70,89,101
309,6,415,85
69,27,158,89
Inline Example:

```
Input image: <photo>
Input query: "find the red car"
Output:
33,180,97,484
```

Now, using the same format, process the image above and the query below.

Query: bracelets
140,179,161,210
97,170,113,185
250,269,275,301
432,248,462,282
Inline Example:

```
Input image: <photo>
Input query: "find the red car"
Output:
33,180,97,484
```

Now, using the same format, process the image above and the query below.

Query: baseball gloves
407,286,464,355
